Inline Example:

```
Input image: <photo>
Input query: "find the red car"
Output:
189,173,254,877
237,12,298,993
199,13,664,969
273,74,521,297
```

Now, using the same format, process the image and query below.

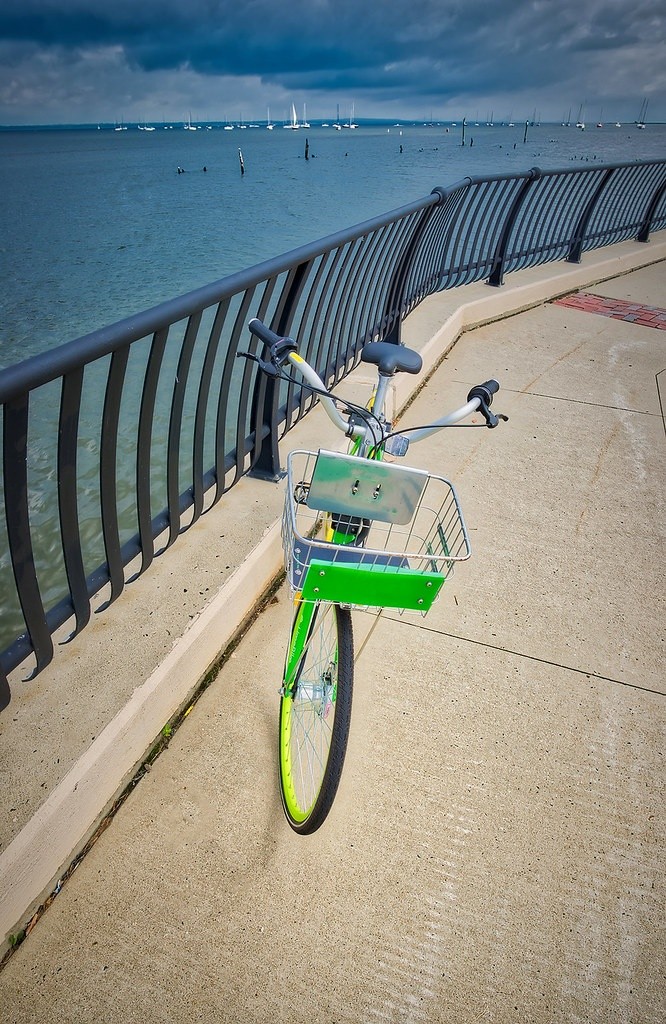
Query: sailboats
508,108,515,127
249,111,260,128
283,102,310,129
474,109,480,127
239,111,247,129
423,107,441,127
462,114,467,126
183,109,197,131
224,111,234,131
561,103,585,127
527,108,541,126
322,96,359,131
486,108,494,126
137,108,156,132
113,112,128,131
634,97,650,129
597,106,603,127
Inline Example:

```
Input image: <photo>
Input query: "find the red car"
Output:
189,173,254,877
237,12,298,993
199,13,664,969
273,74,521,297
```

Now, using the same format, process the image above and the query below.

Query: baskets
281,450,470,617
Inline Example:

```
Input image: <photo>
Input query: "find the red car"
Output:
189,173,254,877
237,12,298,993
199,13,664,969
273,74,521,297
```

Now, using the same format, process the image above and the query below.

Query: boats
394,123,404,127
451,122,457,127
615,121,621,128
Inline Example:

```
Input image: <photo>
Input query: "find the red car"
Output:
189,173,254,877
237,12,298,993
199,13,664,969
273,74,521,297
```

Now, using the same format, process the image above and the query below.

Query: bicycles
236,318,509,836
